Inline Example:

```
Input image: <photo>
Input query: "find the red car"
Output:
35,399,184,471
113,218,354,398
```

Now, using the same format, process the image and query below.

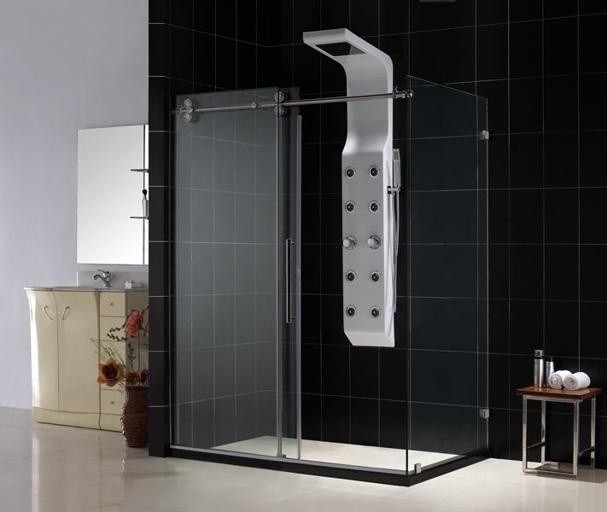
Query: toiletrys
531,349,544,388
544,355,557,387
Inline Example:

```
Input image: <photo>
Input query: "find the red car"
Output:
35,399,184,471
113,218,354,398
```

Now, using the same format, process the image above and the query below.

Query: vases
122,386,150,448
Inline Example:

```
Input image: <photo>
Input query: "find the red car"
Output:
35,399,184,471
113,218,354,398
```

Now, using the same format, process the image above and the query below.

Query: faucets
93,268,111,286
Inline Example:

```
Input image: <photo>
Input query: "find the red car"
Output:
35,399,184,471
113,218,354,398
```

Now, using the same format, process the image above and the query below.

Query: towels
562,372,591,391
549,370,571,389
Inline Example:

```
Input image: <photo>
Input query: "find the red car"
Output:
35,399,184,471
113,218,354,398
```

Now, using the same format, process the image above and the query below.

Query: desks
517,386,601,478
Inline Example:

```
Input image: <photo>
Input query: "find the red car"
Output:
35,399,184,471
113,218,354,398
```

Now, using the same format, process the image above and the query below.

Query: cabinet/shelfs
26,290,99,430
97,293,149,433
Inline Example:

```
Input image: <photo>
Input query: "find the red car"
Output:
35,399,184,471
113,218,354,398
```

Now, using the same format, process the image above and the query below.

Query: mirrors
77,124,150,265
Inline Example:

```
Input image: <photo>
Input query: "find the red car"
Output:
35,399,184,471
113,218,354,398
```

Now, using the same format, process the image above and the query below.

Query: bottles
533,348,554,388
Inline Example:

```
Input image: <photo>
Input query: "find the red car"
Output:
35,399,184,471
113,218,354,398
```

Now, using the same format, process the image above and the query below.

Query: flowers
90,305,151,388
